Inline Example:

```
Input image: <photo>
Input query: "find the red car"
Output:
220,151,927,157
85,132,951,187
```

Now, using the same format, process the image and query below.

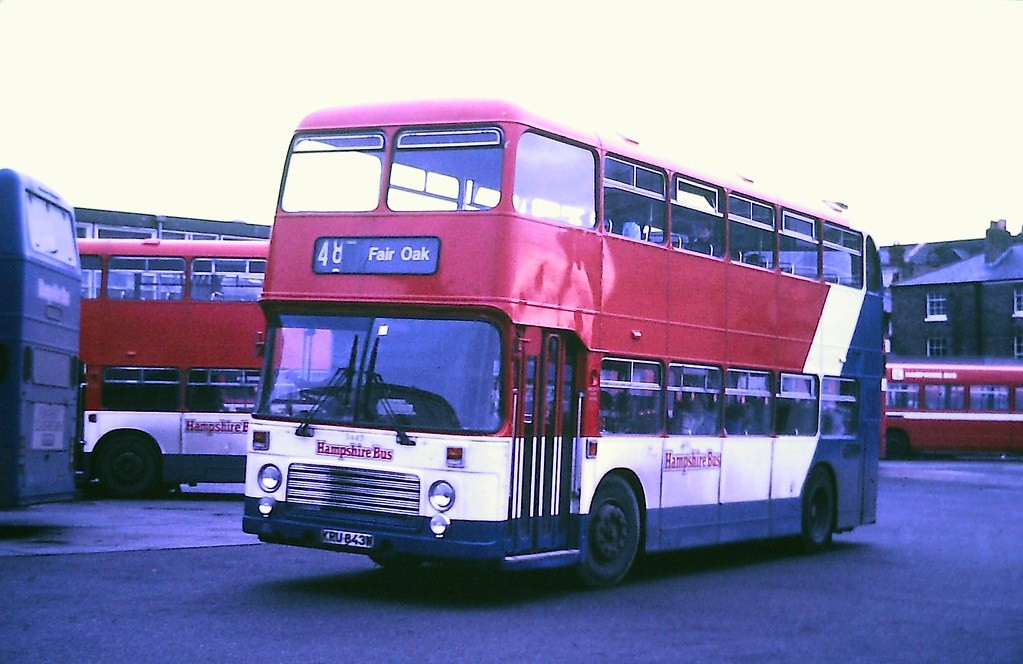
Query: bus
880,361,1023,464
0,165,83,511
235,95,886,594
69,232,333,503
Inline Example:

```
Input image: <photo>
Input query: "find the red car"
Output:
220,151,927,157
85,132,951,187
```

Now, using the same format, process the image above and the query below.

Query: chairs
98,287,261,302
594,385,835,437
594,218,849,288
